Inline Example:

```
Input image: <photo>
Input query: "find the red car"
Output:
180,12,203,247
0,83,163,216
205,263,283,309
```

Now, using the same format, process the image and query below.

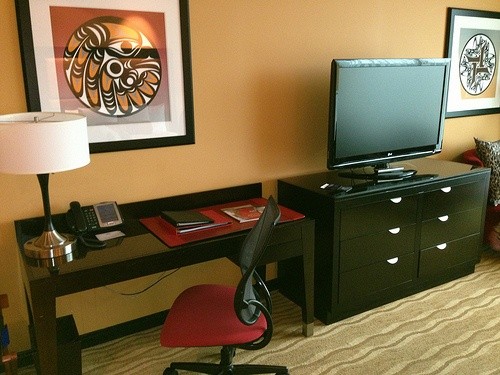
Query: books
220,203,261,223
160,209,212,227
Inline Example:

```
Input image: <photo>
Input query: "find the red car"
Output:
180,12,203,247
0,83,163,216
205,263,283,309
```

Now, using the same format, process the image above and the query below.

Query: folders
161,210,214,228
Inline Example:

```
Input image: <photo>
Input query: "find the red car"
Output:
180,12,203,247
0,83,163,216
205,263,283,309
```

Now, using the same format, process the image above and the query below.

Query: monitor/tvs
326,58,452,182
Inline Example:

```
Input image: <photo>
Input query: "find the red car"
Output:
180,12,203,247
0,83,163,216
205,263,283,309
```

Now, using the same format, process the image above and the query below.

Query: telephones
64,201,124,235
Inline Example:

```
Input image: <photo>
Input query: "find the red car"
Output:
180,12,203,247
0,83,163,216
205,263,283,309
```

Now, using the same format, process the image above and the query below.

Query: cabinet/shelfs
277,157,491,325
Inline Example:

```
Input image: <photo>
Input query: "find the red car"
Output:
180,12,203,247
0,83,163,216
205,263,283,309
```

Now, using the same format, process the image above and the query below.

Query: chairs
463,148,500,243
159,194,290,375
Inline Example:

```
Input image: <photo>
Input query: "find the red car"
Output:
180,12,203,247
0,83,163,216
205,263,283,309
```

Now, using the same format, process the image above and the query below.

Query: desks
14,183,315,375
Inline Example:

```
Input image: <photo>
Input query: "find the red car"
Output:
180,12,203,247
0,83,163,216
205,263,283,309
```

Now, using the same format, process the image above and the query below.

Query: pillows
472,138,500,206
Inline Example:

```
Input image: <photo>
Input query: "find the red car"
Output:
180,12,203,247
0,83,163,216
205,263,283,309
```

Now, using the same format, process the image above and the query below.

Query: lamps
0,112,91,260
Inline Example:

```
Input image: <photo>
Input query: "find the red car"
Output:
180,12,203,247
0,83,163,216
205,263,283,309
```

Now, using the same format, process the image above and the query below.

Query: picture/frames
443,7,500,118
15,0,195,153
